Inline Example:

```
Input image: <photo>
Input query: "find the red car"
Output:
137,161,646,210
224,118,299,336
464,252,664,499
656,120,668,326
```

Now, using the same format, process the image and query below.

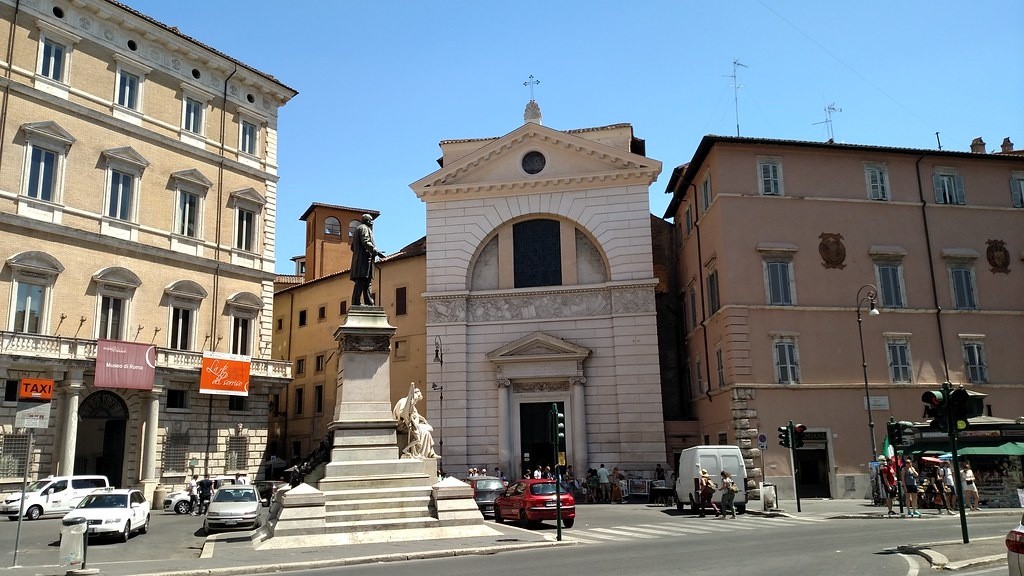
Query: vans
671,444,749,515
0,474,110,521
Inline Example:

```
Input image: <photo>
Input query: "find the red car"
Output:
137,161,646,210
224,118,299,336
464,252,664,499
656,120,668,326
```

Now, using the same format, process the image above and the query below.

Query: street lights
433,335,445,476
856,284,886,507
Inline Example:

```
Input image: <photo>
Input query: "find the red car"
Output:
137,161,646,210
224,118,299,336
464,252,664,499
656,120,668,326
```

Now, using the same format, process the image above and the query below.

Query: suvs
162,475,253,515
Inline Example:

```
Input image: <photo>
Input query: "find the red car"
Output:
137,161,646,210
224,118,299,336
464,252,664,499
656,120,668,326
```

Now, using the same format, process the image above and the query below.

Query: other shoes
970,507,975,510
974,507,981,511
190,512,197,516
715,512,721,517
699,515,706,518
908,510,913,515
938,512,943,514
584,499,628,504
913,510,921,515
720,516,727,520
888,511,898,515
730,516,736,519
947,510,955,514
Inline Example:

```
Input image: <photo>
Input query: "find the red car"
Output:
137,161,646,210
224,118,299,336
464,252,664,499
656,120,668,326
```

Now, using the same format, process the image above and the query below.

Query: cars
248,479,292,508
62,486,151,543
202,484,267,535
493,478,576,530
459,476,508,513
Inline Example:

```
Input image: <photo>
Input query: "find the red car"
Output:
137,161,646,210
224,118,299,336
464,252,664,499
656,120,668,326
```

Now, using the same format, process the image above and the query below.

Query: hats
699,468,709,477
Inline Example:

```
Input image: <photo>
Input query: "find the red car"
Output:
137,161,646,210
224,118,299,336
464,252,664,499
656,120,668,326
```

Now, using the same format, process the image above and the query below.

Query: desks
652,487,673,506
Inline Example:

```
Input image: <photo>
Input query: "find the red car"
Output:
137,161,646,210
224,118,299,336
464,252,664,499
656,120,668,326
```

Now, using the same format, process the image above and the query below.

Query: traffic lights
792,424,807,448
887,422,902,445
553,403,564,445
778,425,792,448
921,388,950,433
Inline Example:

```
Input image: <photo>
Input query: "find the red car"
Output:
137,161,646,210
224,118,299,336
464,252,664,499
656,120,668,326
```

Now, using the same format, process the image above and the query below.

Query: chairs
221,492,233,499
243,492,251,497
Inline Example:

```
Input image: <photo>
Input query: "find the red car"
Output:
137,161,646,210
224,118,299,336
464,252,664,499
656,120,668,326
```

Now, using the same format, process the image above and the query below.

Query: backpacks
726,478,739,493
706,478,716,493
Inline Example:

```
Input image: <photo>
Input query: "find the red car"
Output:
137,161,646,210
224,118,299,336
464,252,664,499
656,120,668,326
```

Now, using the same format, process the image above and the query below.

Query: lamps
73,315,86,339
53,313,67,335
200,333,211,352
213,335,223,352
133,325,144,340
151,327,161,343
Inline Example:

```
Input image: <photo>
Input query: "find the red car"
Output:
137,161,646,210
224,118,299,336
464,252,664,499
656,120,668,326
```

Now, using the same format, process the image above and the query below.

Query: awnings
896,439,1024,455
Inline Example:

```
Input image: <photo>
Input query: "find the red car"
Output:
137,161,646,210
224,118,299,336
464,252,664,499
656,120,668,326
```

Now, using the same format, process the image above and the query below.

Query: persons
719,471,737,519
654,464,665,481
961,465,982,511
188,475,199,516
236,472,244,492
469,467,506,479
392,387,442,458
669,473,677,488
697,468,720,518
350,214,387,306
932,460,957,515
437,469,447,482
901,460,921,515
882,458,898,515
583,464,627,503
523,464,577,500
199,474,215,516
266,484,272,506
